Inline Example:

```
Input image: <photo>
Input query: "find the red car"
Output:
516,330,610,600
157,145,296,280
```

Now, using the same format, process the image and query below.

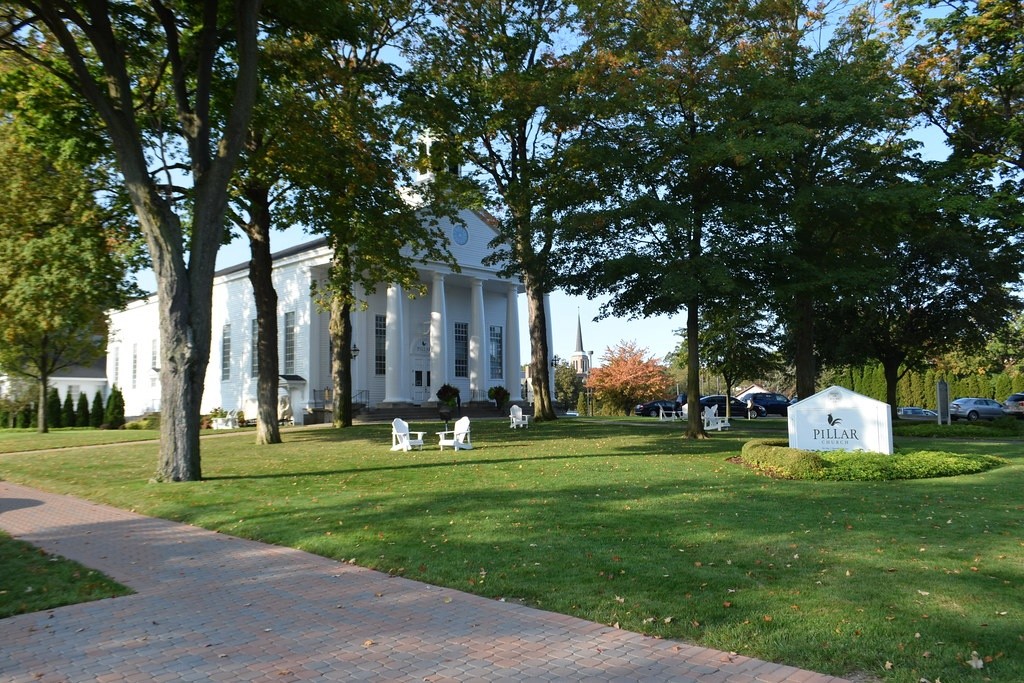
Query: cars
1002,392,1024,417
699,395,767,420
896,406,938,418
634,399,677,417
949,397,1006,421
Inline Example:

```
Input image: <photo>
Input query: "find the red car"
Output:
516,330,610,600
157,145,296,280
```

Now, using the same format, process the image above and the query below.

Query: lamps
550,355,559,367
351,344,359,360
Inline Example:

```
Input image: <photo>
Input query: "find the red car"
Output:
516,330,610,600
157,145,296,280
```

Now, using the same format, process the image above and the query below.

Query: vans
674,394,706,418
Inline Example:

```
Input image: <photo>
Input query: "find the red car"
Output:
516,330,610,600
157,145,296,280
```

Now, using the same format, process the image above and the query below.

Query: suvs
741,392,792,417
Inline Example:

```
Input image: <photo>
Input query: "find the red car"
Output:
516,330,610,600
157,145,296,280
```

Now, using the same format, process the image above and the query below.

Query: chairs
704,406,730,431
678,404,689,422
701,404,718,423
436,417,472,451
658,403,677,422
391,418,427,453
509,405,530,429
